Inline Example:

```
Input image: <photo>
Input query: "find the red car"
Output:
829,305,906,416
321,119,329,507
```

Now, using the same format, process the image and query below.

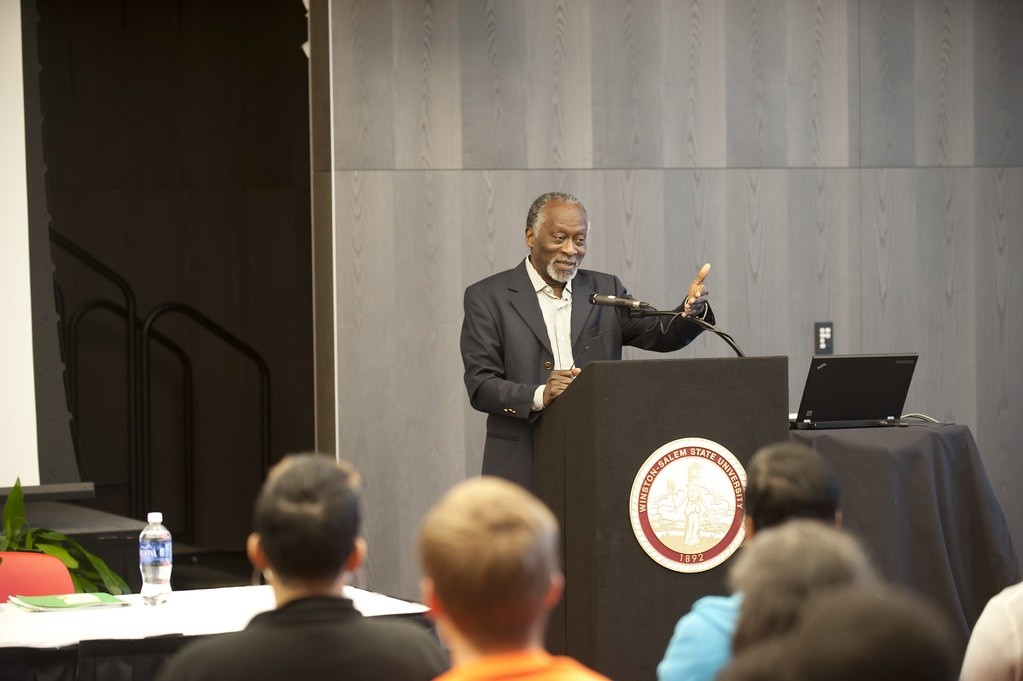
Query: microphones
588,293,649,307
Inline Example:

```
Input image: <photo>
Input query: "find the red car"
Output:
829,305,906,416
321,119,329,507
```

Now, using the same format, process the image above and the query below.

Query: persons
655,438,843,681
716,518,952,681
153,452,451,681
460,192,711,494
957,578,1023,681
416,474,615,681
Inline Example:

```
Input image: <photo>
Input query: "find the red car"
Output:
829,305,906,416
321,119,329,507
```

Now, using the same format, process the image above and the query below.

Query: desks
0,584,436,681
0,493,173,681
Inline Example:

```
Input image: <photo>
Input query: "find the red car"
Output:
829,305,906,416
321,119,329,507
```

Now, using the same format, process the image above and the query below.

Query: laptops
790,352,918,430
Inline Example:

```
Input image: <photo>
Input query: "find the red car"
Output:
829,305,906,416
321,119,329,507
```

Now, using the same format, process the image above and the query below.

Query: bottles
138,512,173,610
